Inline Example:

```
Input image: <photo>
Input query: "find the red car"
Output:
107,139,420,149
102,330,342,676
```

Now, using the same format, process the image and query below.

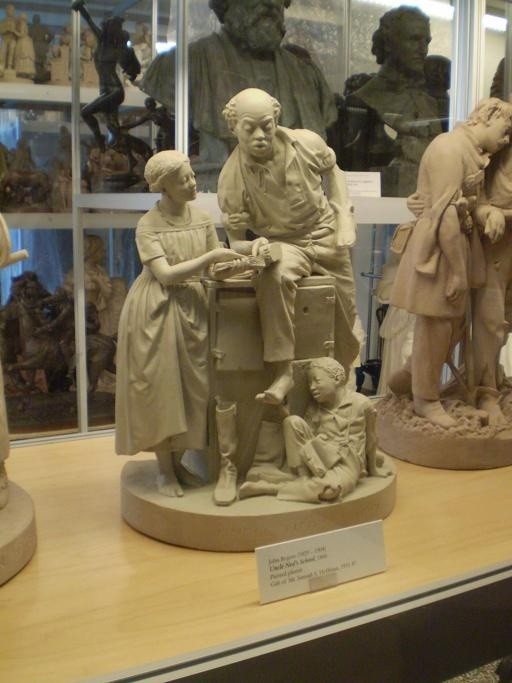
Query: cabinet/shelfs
1,75,164,238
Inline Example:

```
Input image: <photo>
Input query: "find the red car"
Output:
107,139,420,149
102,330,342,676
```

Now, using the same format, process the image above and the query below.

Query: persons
218,87,362,404
394,96,512,430
29,234,127,387
0,0,175,188
342,54,505,172
340,5,443,197
115,150,245,497
239,358,394,504
0,213,29,491
130,0,339,165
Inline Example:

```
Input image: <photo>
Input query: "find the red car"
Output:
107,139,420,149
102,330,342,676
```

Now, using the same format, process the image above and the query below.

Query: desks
4,430,512,681
